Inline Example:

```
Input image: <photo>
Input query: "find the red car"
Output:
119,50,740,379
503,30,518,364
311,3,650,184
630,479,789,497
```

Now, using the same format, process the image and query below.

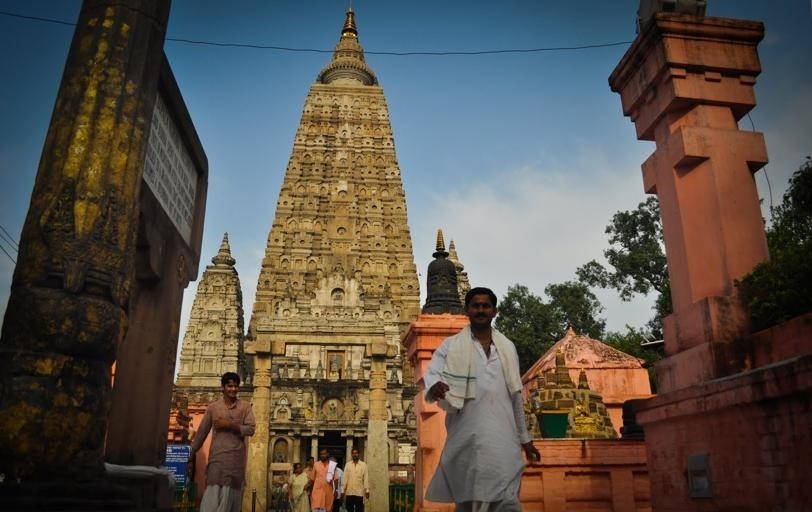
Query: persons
285,446,343,512
186,372,255,512
341,448,369,511
424,286,541,511
